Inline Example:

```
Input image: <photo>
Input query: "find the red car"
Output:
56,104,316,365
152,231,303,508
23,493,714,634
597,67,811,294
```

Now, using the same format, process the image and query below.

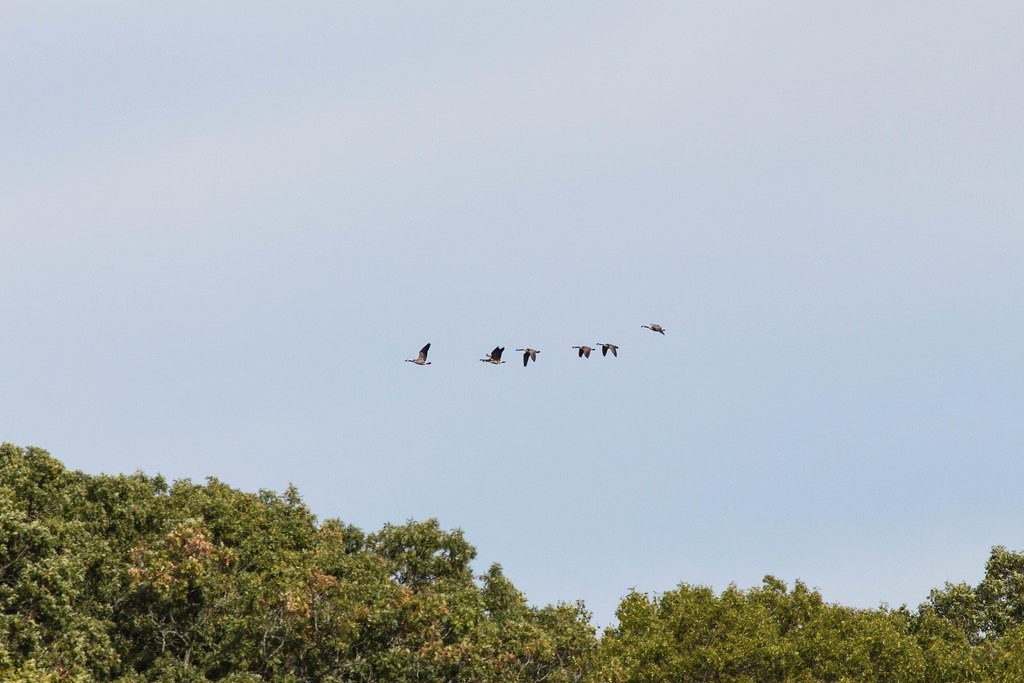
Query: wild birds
479,345,506,368
597,342,619,358
403,342,433,365
571,344,596,358
514,346,541,366
641,323,667,336
